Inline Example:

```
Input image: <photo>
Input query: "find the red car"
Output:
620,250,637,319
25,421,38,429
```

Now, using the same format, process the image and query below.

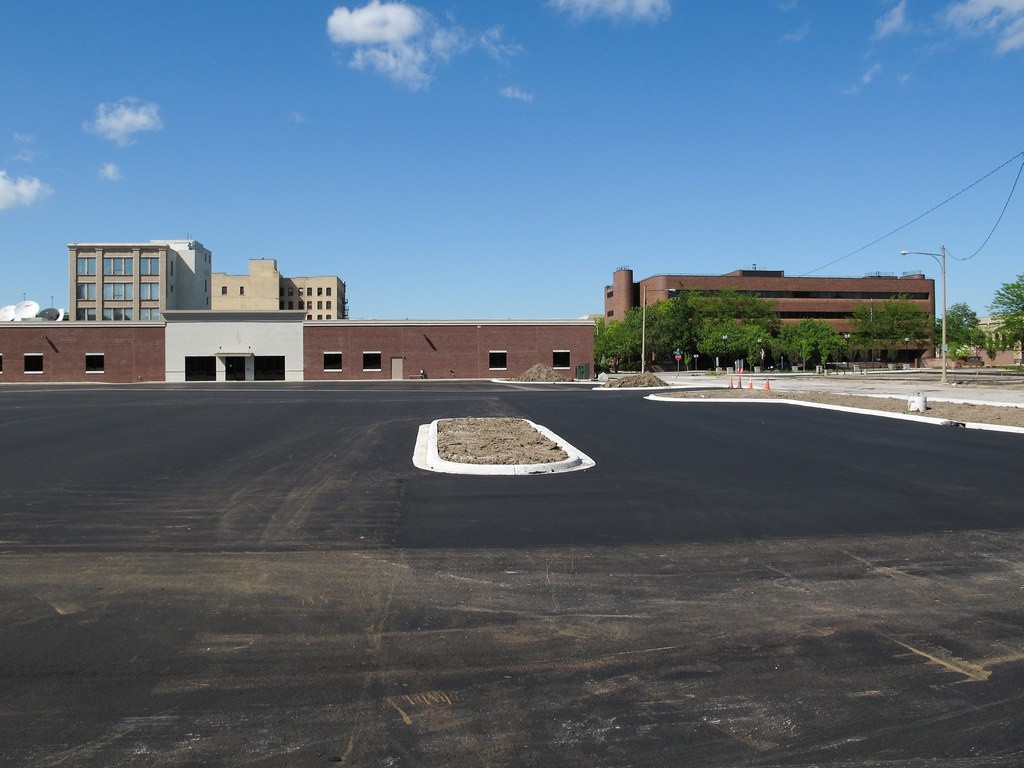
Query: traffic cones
748,377,753,389
764,377,770,390
736,377,742,388
728,376,733,389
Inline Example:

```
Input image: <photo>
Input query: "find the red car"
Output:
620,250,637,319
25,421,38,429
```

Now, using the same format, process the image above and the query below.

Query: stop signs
675,354,681,360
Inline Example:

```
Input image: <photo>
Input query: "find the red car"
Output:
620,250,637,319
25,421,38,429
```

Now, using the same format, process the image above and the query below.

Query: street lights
722,335,728,371
845,333,851,369
901,246,948,385
642,285,676,373
905,337,909,364
757,338,763,372
694,354,699,370
